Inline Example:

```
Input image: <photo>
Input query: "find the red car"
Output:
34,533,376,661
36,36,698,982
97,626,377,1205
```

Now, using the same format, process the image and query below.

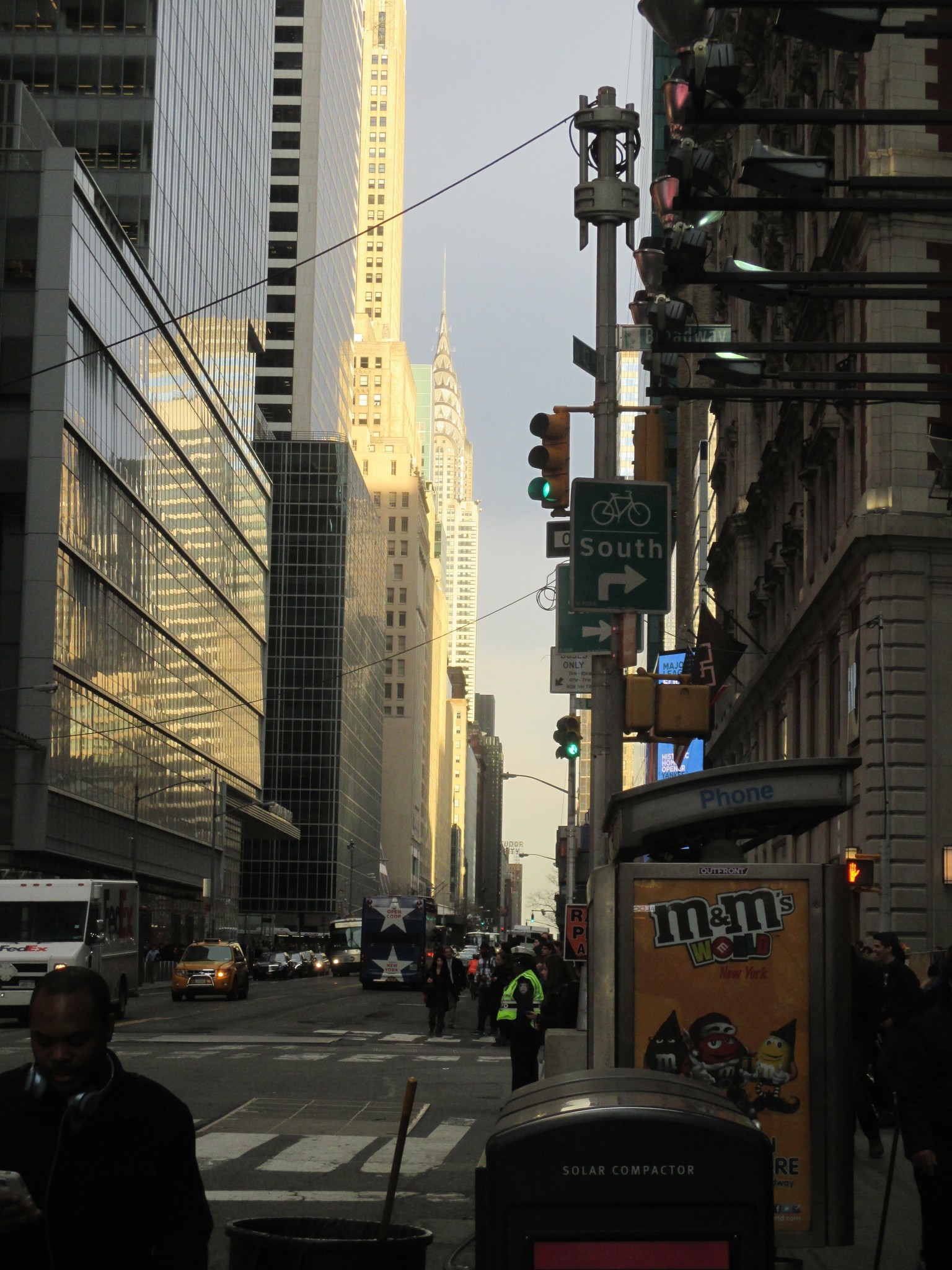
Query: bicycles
469,977,478,1000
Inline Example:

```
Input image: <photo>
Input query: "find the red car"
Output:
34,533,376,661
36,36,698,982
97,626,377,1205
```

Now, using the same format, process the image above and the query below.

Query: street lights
132,755,211,876
500,773,575,906
348,839,389,919
211,769,275,941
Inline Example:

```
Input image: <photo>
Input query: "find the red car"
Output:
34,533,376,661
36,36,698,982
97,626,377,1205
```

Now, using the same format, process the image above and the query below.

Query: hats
511,945,537,958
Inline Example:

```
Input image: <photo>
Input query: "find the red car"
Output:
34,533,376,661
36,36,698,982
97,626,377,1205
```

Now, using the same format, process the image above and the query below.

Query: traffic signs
543,521,574,558
550,646,594,693
557,564,646,652
572,478,673,615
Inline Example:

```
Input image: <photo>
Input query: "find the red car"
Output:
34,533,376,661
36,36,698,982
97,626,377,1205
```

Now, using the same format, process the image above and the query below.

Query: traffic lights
531,915,534,920
631,413,665,482
845,858,876,887
563,715,582,758
528,409,572,509
554,717,565,759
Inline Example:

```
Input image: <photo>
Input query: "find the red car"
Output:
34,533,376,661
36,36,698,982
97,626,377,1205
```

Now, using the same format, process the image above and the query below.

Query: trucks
1,879,141,1024
505,927,558,956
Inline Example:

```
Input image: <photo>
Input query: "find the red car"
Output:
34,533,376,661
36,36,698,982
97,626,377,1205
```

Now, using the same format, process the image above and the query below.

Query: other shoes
868,1140,885,1158
429,1024,498,1038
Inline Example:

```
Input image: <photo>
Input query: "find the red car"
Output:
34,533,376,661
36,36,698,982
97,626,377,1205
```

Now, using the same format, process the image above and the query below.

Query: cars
286,953,306,977
253,952,295,981
314,952,330,976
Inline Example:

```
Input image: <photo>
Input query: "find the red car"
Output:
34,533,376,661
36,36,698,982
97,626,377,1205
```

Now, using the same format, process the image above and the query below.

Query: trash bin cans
224,1214,433,1270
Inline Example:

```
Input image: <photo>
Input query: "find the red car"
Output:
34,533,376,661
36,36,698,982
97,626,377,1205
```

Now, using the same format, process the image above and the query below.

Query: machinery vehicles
237,927,330,952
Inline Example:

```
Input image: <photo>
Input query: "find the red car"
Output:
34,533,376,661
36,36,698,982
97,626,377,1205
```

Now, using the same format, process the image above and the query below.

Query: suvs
298,950,318,976
172,939,249,1004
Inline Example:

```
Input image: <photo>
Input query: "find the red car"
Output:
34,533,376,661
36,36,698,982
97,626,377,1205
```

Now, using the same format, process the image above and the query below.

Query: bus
361,894,437,989
456,932,502,967
330,919,362,977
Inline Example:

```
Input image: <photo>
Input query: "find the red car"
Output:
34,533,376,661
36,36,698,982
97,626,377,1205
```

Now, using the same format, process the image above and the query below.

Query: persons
534,955,577,1062
487,932,576,1030
422,953,460,1038
859,929,924,1158
0,965,214,1269
899,946,952,1270
439,946,467,1029
466,953,480,1000
138,935,185,984
470,942,500,1036
850,924,886,1161
496,945,549,1093
